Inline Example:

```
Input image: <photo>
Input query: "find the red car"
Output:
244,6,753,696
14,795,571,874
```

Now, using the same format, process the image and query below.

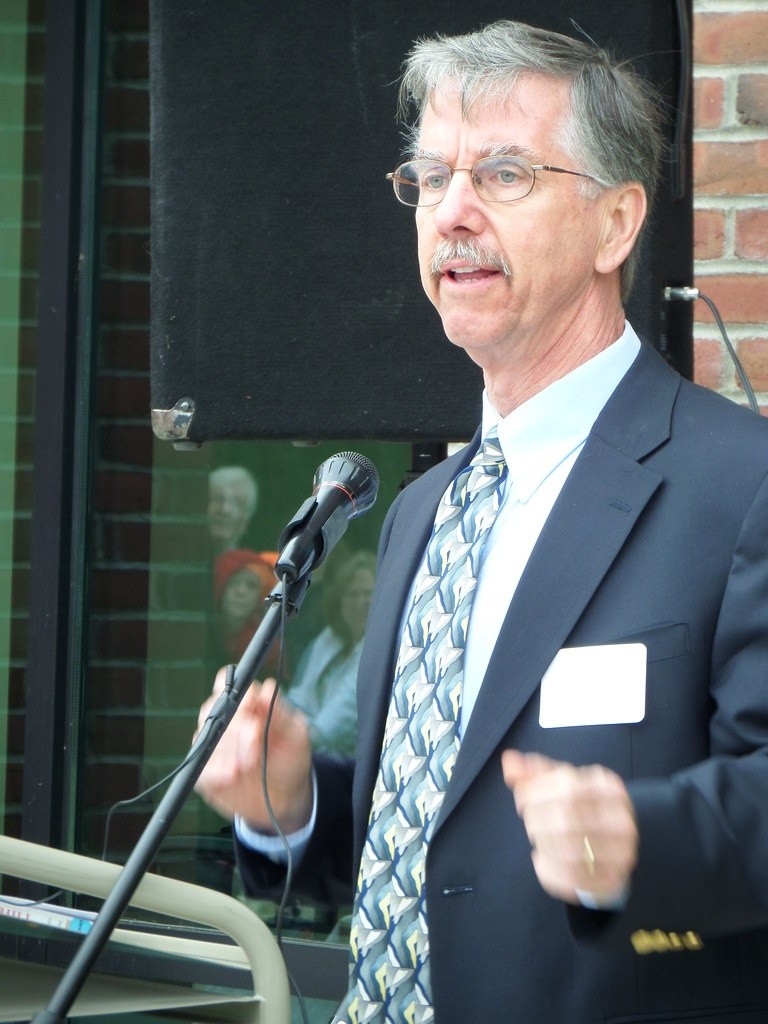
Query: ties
330,424,508,1021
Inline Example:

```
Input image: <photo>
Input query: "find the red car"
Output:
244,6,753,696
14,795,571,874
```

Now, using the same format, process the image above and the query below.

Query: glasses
386,156,621,207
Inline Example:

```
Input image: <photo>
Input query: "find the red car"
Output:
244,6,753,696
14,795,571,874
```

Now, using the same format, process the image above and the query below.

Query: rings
583,831,607,872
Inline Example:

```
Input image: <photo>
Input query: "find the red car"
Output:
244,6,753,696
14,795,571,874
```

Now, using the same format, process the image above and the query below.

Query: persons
284,552,381,760
212,546,288,683
207,466,256,553
188,21,768,1024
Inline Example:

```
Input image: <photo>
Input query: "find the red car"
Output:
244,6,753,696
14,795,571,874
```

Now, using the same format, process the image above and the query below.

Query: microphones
273,450,380,586
